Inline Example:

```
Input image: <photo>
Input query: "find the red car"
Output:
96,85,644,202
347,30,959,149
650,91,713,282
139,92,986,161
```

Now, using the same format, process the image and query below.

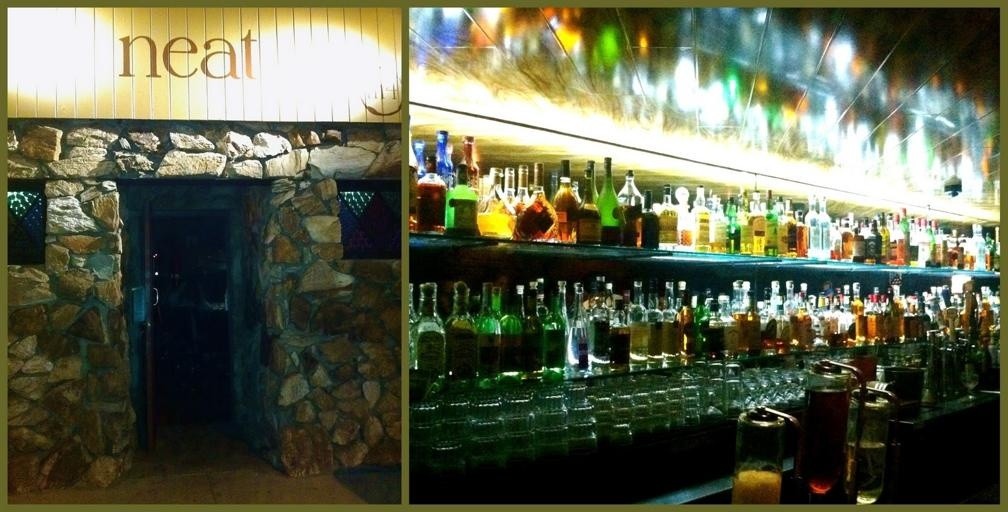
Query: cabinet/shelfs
409,232,1000,504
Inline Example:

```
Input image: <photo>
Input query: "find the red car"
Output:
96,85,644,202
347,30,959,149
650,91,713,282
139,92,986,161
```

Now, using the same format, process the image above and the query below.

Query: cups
717,360,815,406
891,348,997,400
406,373,727,463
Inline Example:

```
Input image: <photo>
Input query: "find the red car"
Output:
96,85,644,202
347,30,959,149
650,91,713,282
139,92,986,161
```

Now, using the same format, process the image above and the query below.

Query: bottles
408,125,673,247
728,398,800,505
844,383,901,501
674,181,1000,269
796,355,869,504
406,275,1001,373
